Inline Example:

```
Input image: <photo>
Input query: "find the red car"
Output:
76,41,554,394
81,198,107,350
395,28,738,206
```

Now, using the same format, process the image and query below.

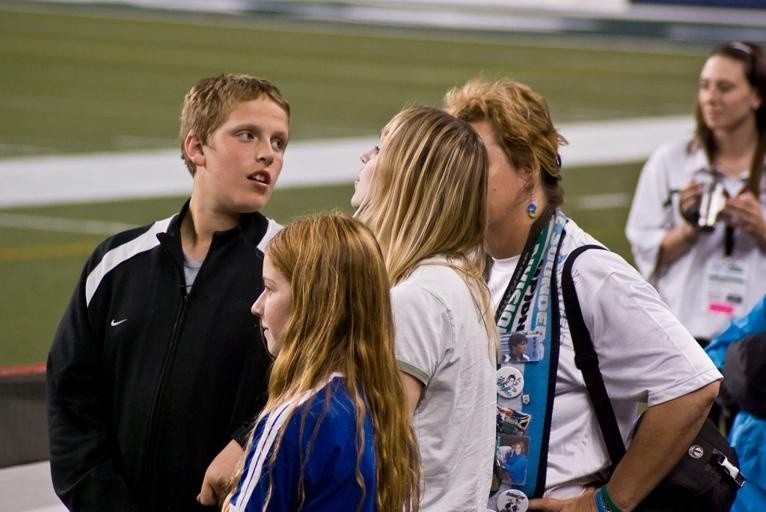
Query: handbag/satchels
631,411,746,512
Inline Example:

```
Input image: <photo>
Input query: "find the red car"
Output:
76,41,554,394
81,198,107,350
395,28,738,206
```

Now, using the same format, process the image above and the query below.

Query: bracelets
592,484,620,512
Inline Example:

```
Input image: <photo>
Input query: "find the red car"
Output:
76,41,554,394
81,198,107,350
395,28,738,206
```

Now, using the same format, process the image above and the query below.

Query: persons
499,372,517,392
441,76,725,511
214,208,430,511
700,279,763,511
341,103,503,510
503,332,532,363
496,437,525,486
43,73,291,511
621,38,764,437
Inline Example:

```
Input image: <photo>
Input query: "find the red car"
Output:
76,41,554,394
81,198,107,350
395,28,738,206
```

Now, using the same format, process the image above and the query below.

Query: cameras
692,183,730,233
